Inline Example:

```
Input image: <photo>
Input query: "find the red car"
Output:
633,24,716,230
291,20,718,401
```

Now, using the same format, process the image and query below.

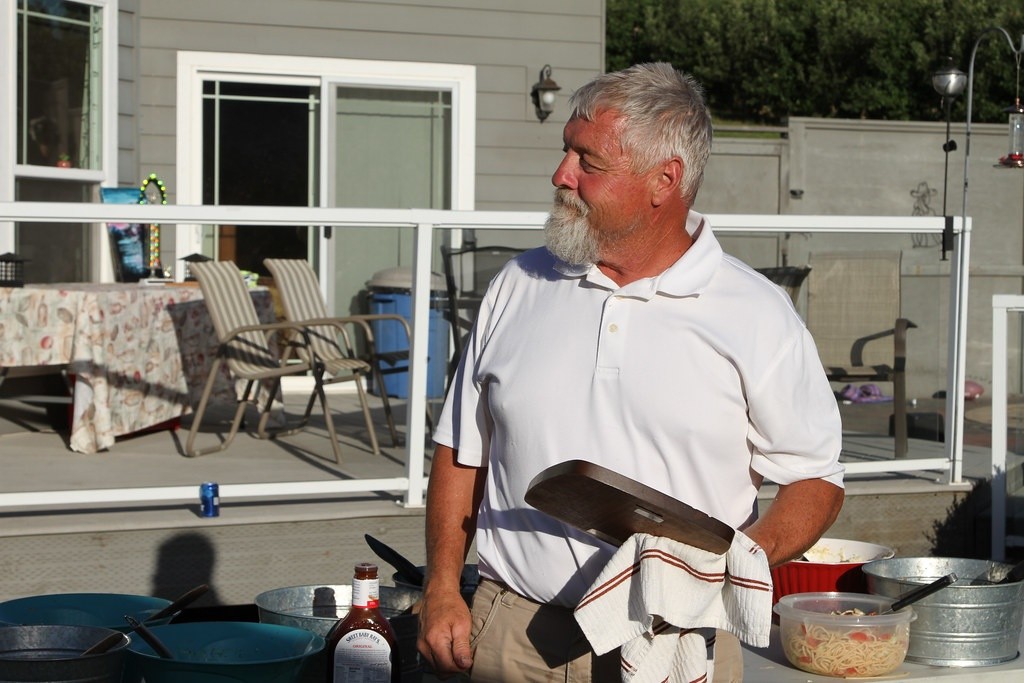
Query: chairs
806,249,918,456
262,258,438,462
430,245,527,399
183,260,380,465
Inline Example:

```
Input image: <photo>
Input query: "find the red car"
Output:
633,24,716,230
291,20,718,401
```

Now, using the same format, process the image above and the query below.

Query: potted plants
54,152,74,167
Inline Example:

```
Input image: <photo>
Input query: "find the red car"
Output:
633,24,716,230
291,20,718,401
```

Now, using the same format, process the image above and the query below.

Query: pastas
788,609,899,676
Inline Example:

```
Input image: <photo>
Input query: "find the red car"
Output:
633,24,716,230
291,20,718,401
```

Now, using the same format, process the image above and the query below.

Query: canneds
199,481,219,516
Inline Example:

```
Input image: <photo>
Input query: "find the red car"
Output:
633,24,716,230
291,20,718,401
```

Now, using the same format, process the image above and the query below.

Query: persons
419,62,845,683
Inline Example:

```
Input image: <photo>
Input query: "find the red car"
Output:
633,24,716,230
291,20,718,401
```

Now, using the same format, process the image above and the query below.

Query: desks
740,625,1024,683
0,284,281,453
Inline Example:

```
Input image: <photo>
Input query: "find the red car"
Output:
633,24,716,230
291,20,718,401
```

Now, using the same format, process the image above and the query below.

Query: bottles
321,562,403,683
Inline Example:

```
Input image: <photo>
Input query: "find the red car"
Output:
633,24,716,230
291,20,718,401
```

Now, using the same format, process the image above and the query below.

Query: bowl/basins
125,622,326,683
0,622,132,683
254,584,426,683
0,592,182,635
861,556,1024,667
770,537,896,626
392,563,482,608
773,592,918,677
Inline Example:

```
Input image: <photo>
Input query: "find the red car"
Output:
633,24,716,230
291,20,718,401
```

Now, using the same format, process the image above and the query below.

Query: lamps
532,64,562,111
992,95,1024,168
932,67,968,262
